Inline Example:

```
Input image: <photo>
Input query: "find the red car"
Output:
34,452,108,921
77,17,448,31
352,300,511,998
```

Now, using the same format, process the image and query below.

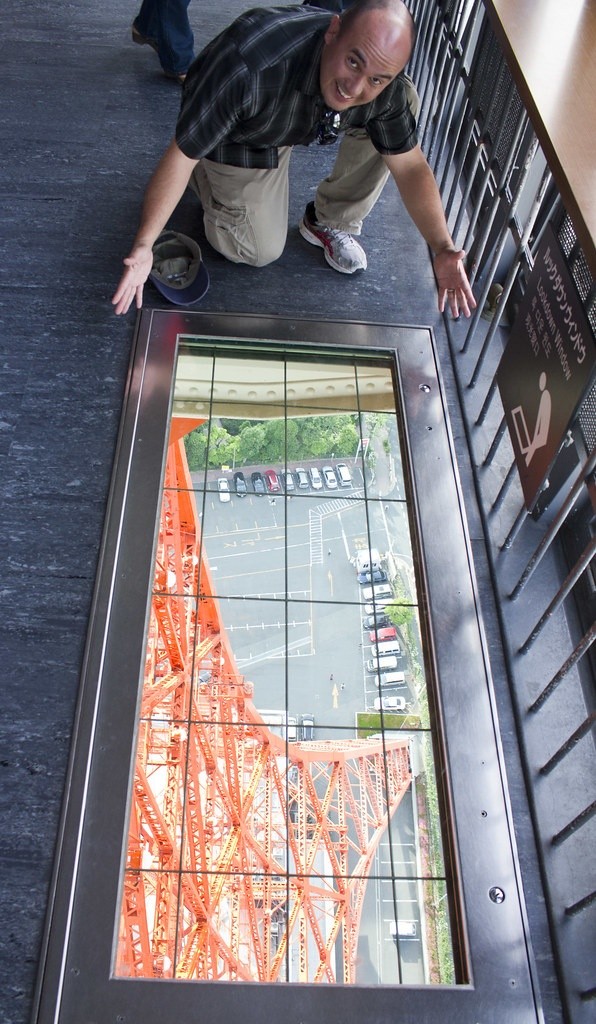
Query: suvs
363,585,392,600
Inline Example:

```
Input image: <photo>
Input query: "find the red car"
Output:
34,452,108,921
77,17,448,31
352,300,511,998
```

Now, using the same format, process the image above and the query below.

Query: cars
365,656,398,673
357,569,388,585
295,467,309,489
280,468,295,491
363,616,389,629
233,472,248,498
369,628,397,643
322,466,338,489
373,696,406,712
250,471,267,497
308,467,323,490
335,463,352,487
299,714,316,742
217,477,231,503
287,717,298,742
265,469,281,492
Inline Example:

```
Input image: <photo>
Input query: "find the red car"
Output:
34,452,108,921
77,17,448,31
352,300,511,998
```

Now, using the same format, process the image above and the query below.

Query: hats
146,230,208,304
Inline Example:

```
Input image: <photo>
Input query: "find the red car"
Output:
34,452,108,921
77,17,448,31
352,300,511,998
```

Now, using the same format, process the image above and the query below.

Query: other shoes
132,24,160,53
163,68,187,84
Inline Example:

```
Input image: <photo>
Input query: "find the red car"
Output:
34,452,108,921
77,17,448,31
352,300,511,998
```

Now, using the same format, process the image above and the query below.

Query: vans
357,559,382,573
390,921,417,937
371,641,401,658
365,598,395,615
374,671,406,688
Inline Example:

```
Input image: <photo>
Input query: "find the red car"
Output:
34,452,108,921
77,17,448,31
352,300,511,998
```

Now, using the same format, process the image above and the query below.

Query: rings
447,290,454,292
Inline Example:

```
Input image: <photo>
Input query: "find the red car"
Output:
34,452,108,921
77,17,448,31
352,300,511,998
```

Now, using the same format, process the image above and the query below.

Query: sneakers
300,204,367,276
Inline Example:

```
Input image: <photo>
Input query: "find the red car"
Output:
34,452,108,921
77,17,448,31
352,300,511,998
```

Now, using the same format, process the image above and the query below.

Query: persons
113,1,479,321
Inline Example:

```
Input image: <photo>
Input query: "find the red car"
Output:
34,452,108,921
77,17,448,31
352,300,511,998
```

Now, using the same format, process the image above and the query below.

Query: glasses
316,102,341,145
